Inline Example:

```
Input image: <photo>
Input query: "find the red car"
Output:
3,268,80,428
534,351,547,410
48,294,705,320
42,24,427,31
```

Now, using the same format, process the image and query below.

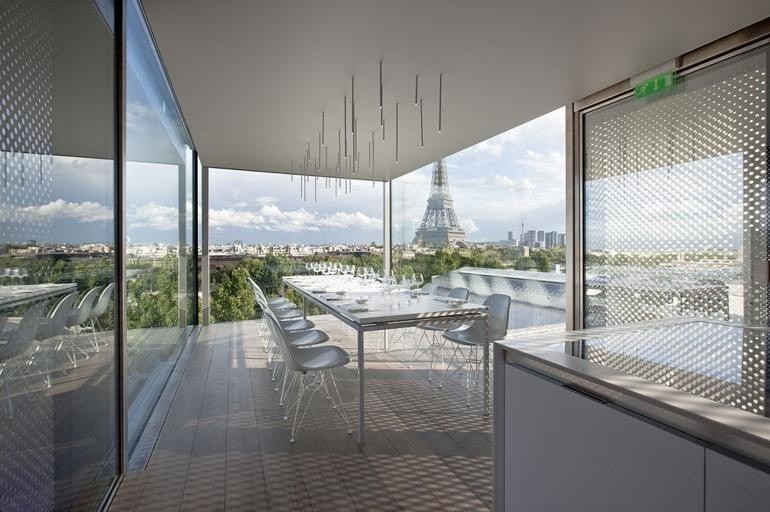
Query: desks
281,274,492,443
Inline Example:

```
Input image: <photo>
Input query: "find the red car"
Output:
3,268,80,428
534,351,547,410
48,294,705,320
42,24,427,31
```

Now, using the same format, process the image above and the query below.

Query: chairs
0,282,114,419
358,271,511,409
245,272,354,437
